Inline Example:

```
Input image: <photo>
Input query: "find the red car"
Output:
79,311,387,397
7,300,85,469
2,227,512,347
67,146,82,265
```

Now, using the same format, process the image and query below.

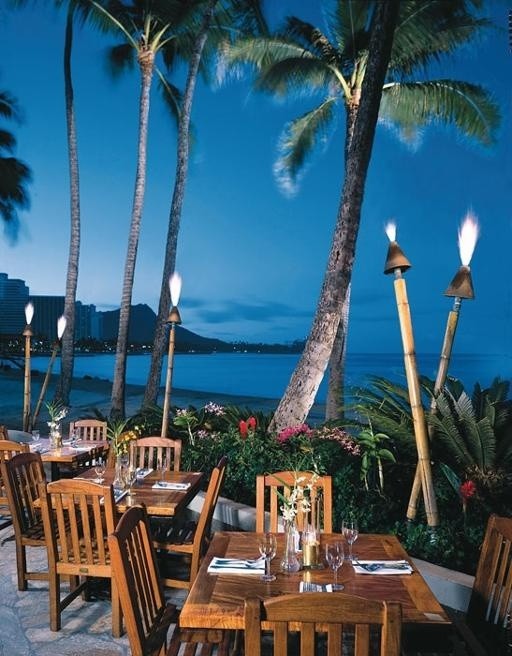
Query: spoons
215,562,251,567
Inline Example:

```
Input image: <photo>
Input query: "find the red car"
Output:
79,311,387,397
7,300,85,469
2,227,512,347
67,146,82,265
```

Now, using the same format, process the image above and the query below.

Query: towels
71,477,104,484
351,558,414,575
152,481,190,490
20,444,37,454
207,555,266,575
99,488,127,505
72,445,95,452
135,467,153,479
62,437,77,445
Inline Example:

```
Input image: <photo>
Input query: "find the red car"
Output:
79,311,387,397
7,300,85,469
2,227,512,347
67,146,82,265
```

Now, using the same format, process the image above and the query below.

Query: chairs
143,457,228,591
107,440,116,468
37,477,139,638
128,436,182,553
58,419,107,477
0,425,11,496
0,450,90,591
106,502,245,655
244,591,403,655
466,512,512,632
0,440,49,514
256,471,333,532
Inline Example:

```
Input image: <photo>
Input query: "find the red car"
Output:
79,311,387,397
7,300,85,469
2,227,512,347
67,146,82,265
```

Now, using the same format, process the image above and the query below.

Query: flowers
46,406,69,435
115,423,145,457
279,473,320,522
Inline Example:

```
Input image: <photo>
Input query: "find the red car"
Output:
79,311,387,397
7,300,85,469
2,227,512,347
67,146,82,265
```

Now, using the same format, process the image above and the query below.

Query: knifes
211,565,266,570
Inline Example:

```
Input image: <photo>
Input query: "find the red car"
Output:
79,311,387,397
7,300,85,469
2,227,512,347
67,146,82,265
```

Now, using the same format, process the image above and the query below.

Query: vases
48,435,60,448
280,524,300,573
112,457,125,489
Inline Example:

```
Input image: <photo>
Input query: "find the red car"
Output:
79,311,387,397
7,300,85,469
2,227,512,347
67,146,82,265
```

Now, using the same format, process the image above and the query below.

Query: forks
217,559,254,563
157,482,186,488
357,562,409,571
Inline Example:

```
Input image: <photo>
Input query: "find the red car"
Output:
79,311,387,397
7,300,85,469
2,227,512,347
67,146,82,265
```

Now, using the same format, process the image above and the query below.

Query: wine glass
70,425,81,446
325,542,344,590
257,534,277,582
302,525,316,544
119,467,139,497
93,457,108,484
156,457,168,485
31,430,40,447
51,421,62,453
341,519,359,563
